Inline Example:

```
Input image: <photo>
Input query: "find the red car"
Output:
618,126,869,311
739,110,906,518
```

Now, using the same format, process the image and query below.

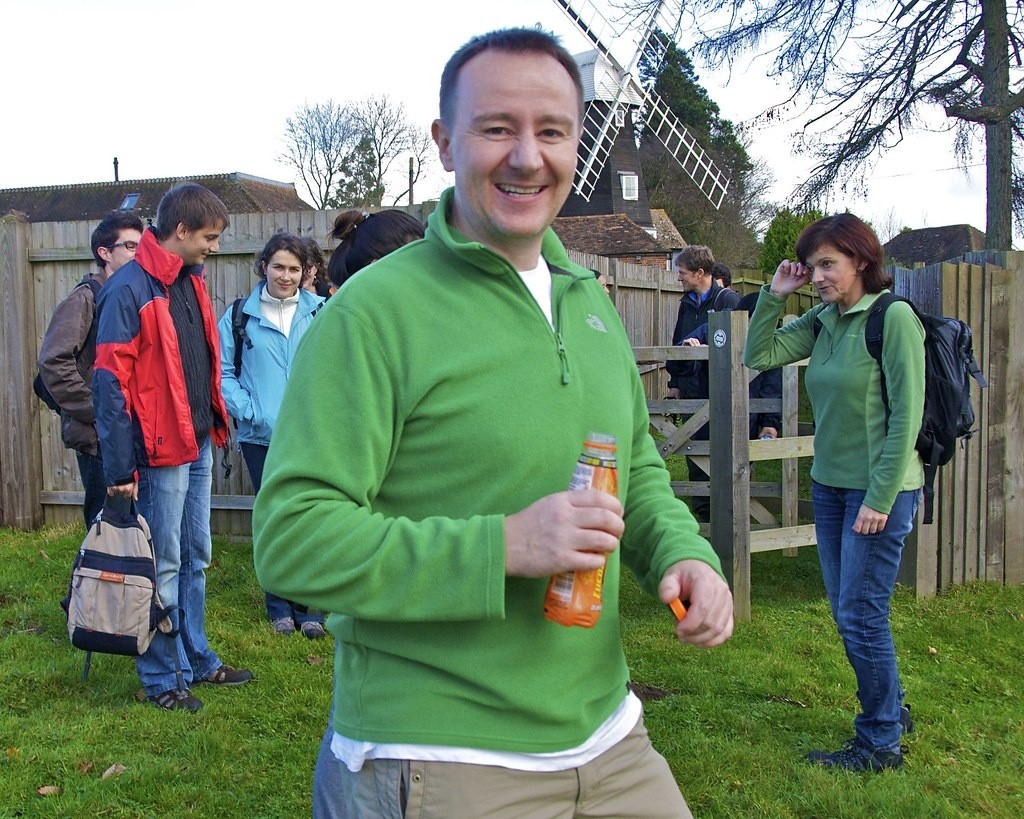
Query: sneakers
806,738,910,774
146,688,203,714
191,664,252,687
301,621,326,639
272,618,295,634
898,702,913,733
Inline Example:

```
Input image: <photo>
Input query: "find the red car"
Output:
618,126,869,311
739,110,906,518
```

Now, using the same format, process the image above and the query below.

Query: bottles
544,433,619,628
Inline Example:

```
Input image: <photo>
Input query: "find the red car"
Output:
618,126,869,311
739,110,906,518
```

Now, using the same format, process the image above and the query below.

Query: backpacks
63,494,185,692
33,279,102,413
814,293,989,524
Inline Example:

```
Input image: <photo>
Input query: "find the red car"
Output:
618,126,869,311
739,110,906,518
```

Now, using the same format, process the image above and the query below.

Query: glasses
108,240,138,252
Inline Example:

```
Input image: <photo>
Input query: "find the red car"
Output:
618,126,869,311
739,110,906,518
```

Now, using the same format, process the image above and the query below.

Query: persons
675,291,782,513
40,214,144,622
667,245,741,523
254,30,734,819
219,210,426,639
744,212,926,774
92,181,251,714
589,268,610,295
